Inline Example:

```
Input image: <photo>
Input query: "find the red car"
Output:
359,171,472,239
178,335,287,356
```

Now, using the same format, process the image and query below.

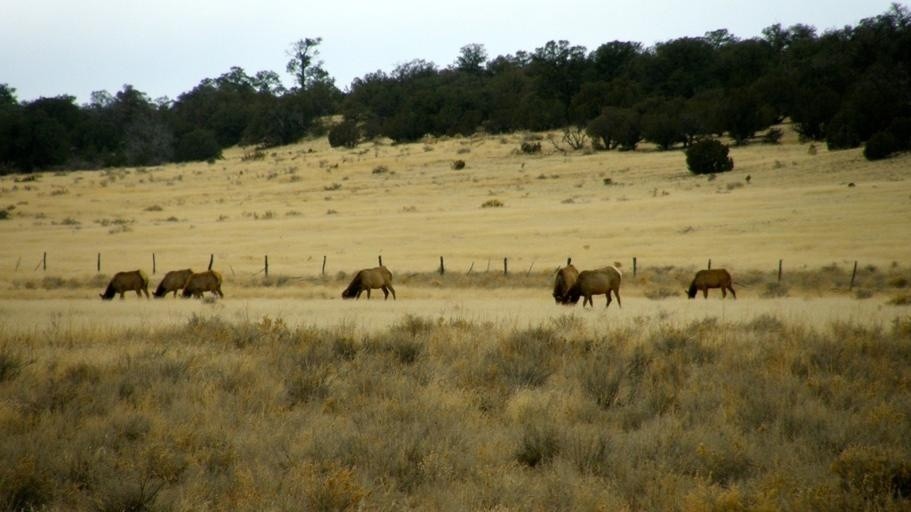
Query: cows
568,265,623,307
152,267,194,299
180,269,224,299
684,268,736,299
552,264,580,305
99,268,149,301
341,265,396,301
